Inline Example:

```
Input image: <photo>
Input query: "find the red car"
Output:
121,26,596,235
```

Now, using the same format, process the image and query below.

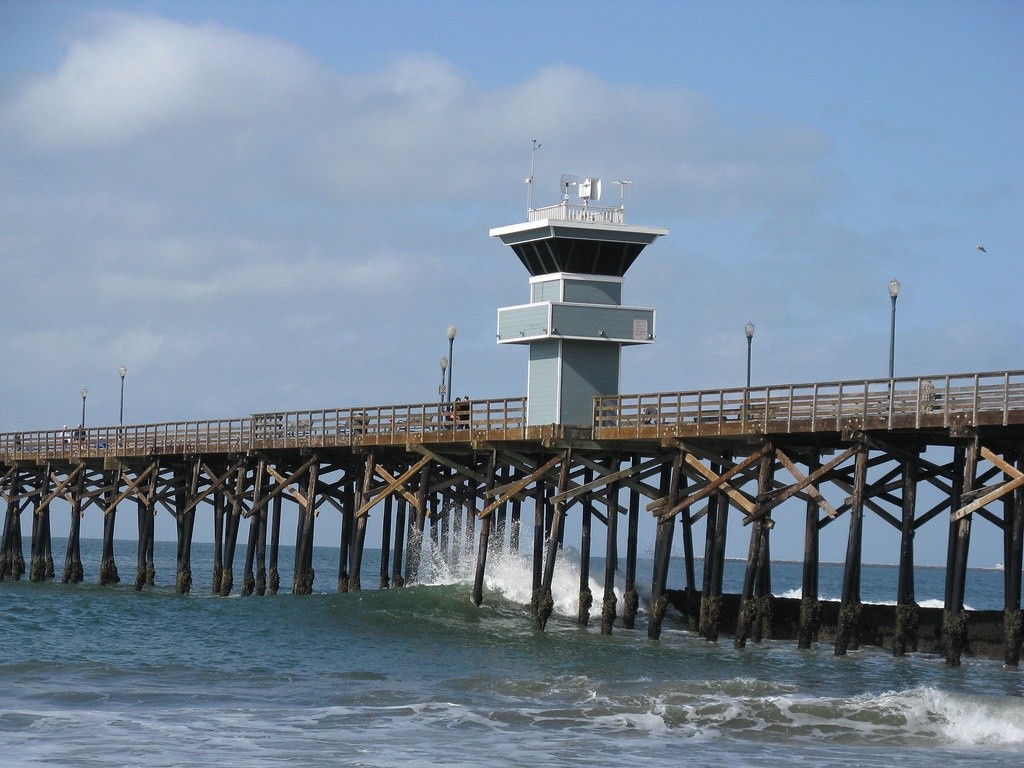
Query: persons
14,423,107,450
451,397,470,429
353,413,370,434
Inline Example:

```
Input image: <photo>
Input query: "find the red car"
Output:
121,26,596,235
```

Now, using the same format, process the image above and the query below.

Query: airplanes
975,245,987,253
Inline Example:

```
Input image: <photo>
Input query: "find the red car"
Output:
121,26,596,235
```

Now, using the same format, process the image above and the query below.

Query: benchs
738,406,779,423
398,414,433,431
283,421,313,437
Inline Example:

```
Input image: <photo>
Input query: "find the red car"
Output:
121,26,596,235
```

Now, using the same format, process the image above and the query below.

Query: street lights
118,365,126,448
439,355,448,429
887,278,900,413
80,387,88,439
745,321,755,419
446,325,457,430
